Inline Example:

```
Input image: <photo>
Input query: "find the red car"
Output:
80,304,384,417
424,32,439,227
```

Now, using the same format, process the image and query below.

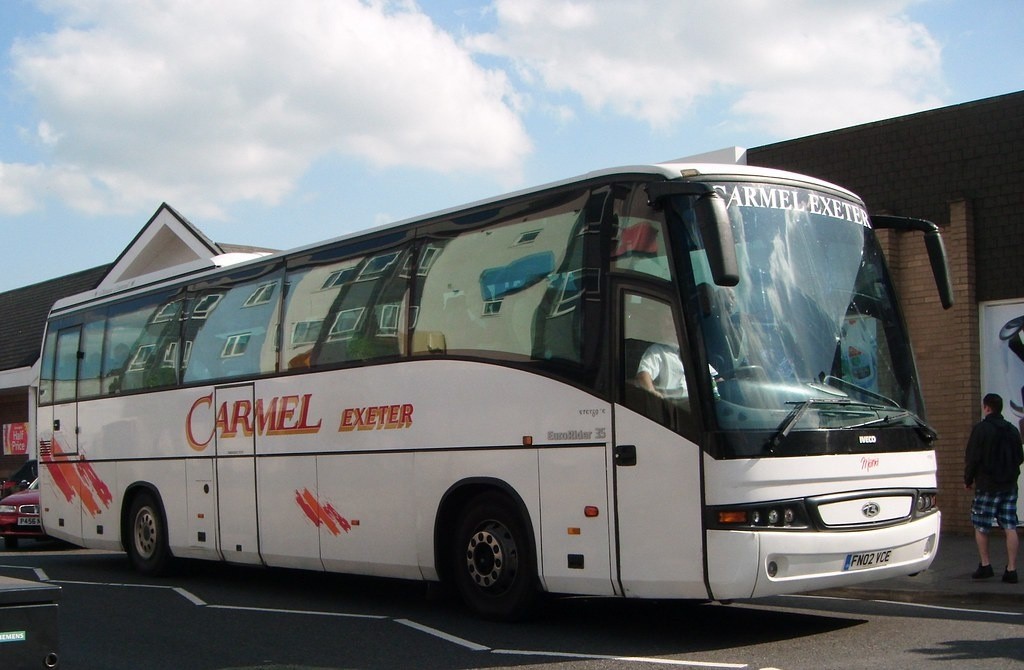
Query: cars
0,459,38,499
0,476,44,540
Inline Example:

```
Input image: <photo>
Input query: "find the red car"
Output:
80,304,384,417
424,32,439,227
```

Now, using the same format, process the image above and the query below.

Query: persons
636,339,723,398
963,393,1024,584
0,480,30,501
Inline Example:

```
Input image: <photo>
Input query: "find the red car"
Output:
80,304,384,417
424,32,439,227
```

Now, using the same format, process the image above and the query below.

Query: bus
36,164,952,621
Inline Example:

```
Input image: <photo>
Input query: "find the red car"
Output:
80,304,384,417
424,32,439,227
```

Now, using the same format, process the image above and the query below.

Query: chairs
626,337,674,383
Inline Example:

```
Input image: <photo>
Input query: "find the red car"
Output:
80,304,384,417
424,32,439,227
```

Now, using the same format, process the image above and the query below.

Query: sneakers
1003,566,1017,583
972,563,994,579
